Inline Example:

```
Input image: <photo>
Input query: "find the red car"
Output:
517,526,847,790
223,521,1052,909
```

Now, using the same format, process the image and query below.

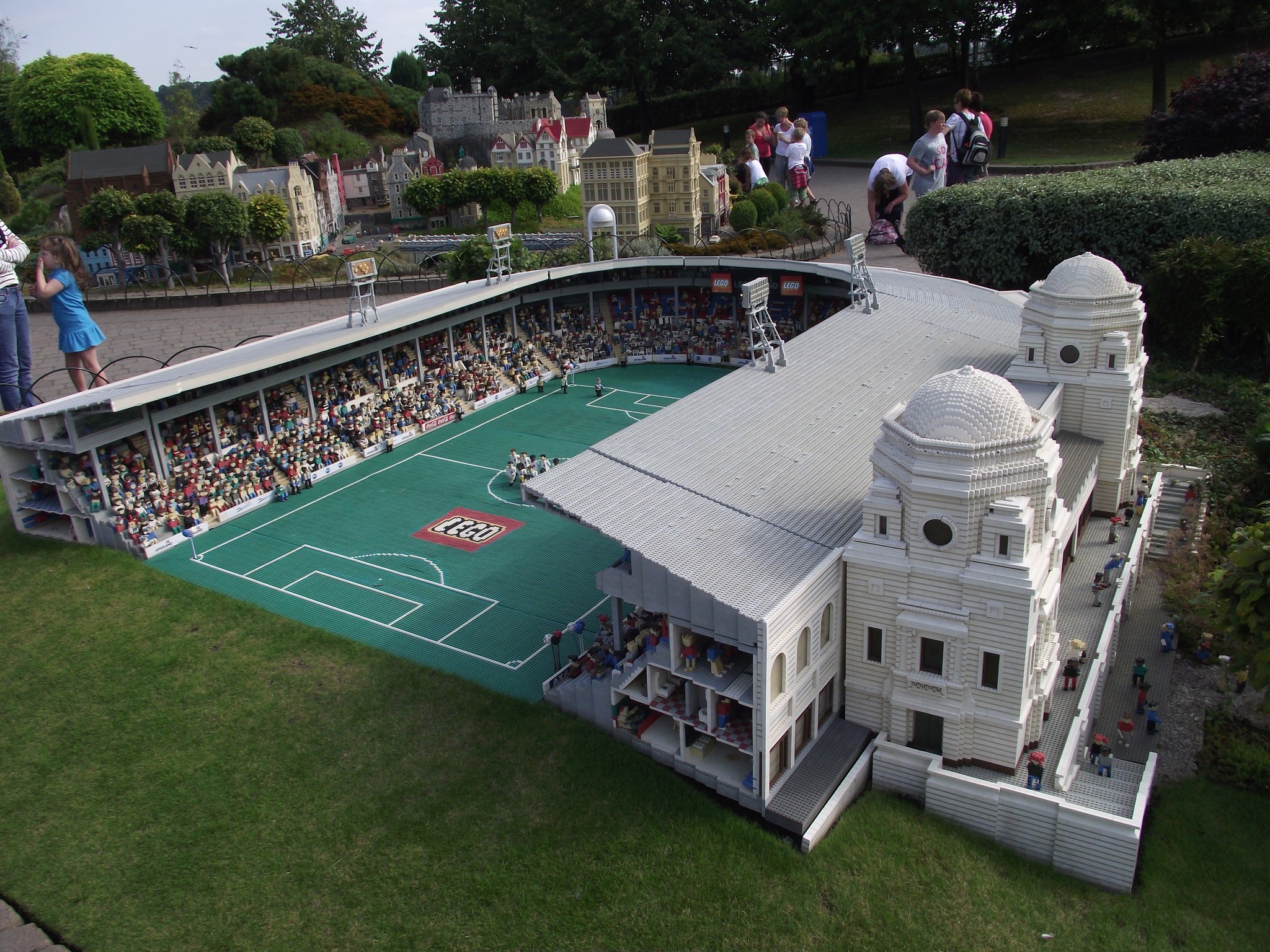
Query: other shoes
808,198,818,207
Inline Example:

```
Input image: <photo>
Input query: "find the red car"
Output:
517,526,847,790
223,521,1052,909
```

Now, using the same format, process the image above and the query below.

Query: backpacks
951,112,989,166
868,216,899,246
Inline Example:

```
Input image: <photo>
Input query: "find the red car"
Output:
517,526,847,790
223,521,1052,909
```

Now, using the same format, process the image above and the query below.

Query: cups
778,132,783,140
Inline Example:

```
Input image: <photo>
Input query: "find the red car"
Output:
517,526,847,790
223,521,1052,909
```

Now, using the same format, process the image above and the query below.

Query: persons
1187,483,1197,509
37,234,107,392
1090,657,1162,778
53,278,493,552
748,110,772,179
744,128,760,163
1091,473,1152,609
738,147,769,192
867,154,913,245
371,238,376,247
774,106,796,192
564,604,745,746
387,232,392,241
1235,664,1249,692
938,88,993,184
506,448,559,502
1196,634,1214,662
356,227,382,238
0,218,31,414
1026,750,1044,793
1062,639,1087,692
1161,622,1176,653
894,111,948,256
785,128,807,207
486,233,859,399
793,118,817,205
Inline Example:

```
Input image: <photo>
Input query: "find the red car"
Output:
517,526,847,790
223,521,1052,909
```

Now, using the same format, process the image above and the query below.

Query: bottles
964,142,969,146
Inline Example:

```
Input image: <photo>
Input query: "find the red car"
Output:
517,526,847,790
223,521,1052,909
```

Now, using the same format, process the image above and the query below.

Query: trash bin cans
796,111,829,159
